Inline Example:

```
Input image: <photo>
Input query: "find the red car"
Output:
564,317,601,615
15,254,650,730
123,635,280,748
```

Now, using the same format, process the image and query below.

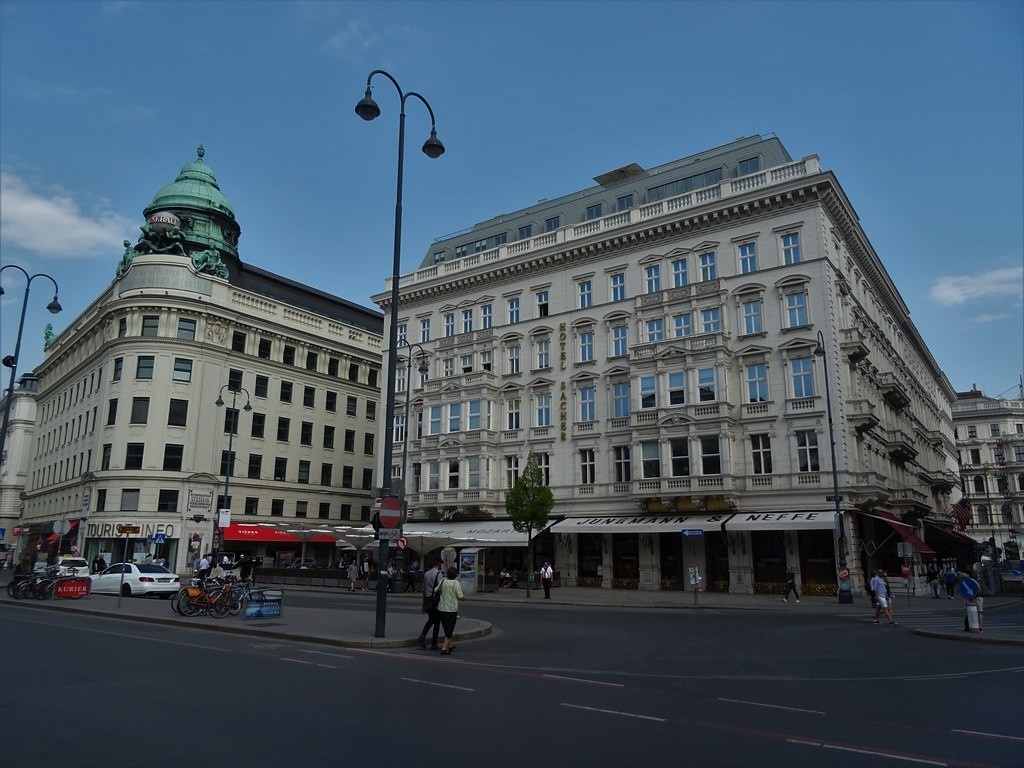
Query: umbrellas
405,535,466,570
264,523,376,567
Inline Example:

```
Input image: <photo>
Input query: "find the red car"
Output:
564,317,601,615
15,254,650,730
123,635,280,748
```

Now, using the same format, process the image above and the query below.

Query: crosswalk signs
154,533,166,544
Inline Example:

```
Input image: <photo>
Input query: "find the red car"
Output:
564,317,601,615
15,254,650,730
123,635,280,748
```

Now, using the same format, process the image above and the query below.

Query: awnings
551,510,844,533
218,523,339,542
853,511,978,558
48,522,79,541
346,520,556,547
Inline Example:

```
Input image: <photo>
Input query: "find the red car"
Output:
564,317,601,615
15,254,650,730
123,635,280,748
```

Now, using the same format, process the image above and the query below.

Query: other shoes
951,597,953,599
889,621,898,625
441,650,451,655
783,599,787,602
873,621,881,624
979,627,983,632
431,645,441,650
948,596,950,599
796,599,800,603
543,596,551,599
449,645,456,650
418,636,426,649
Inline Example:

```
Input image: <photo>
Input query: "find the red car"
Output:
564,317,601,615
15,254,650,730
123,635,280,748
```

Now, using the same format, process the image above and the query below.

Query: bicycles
171,576,267,619
7,566,85,601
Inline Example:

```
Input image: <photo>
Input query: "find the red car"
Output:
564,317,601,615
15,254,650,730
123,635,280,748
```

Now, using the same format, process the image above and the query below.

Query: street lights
0,264,64,468
354,70,445,638
215,384,253,563
813,329,852,606
395,338,430,582
984,464,997,549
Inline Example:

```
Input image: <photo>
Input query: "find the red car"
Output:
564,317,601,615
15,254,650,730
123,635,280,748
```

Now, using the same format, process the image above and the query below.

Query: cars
282,556,317,569
89,560,182,600
48,556,90,578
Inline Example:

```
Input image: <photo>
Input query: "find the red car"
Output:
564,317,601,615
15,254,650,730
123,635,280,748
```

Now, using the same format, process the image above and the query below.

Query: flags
953,497,971,530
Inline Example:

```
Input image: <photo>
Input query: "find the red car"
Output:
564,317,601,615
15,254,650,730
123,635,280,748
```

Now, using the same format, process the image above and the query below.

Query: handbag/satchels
424,596,437,613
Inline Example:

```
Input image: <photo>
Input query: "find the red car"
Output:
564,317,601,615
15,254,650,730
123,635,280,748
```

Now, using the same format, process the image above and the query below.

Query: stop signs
379,497,401,529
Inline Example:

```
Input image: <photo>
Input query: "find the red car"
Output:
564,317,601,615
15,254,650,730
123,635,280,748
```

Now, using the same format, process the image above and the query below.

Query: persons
195,243,221,273
93,554,165,573
869,569,897,624
193,554,259,585
783,566,800,602
540,561,553,598
138,225,186,255
116,241,135,277
418,560,465,654
347,560,418,593
488,568,519,587
926,565,983,631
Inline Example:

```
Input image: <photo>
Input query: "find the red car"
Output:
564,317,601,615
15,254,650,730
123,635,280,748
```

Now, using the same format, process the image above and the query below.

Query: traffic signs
682,528,705,537
